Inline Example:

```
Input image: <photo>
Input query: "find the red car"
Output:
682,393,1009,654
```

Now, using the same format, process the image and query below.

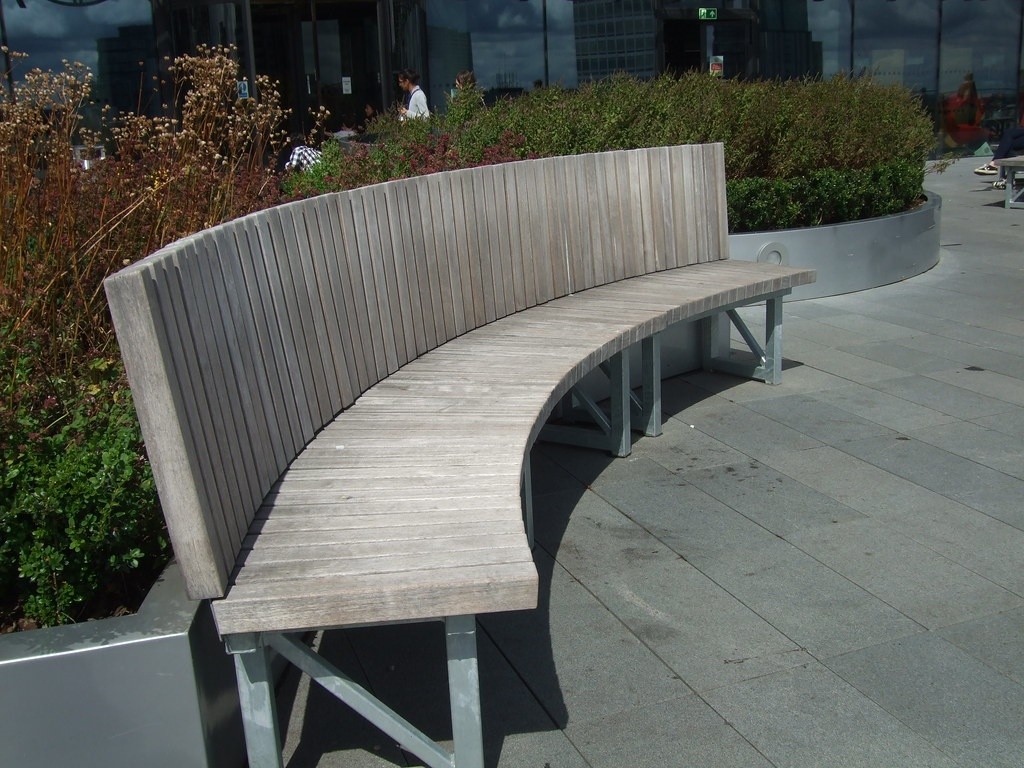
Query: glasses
398,81,404,83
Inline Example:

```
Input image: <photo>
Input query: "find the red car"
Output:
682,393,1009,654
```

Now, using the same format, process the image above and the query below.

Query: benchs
994,155,1024,210
104,139,817,768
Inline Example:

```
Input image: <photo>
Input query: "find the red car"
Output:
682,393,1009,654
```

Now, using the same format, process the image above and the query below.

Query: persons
324,102,377,139
456,70,486,106
974,128,1024,190
285,137,323,171
399,67,430,120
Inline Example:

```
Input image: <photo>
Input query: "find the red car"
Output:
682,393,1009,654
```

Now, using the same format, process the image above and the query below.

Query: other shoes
974,163,998,175
992,177,1016,190
